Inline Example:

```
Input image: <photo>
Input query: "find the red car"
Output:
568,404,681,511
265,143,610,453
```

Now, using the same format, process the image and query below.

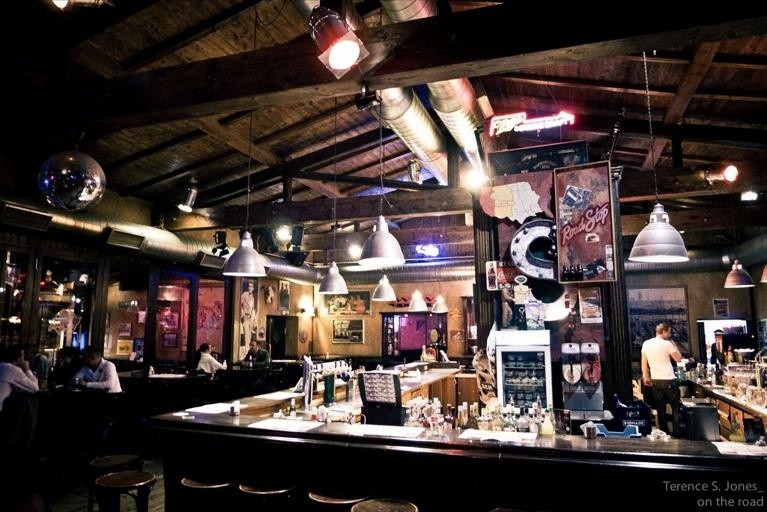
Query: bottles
420,345,426,363
510,402,518,422
726,344,734,363
39,359,47,388
706,359,713,383
583,422,597,439
488,263,497,289
444,404,452,422
540,410,554,438
466,405,478,429
477,408,492,430
248,355,253,371
472,402,480,419
501,404,516,432
562,264,582,282
529,403,544,417
760,356,767,389
489,406,504,431
462,402,468,427
524,404,532,418
517,406,530,432
731,378,737,396
289,398,296,418
449,407,457,428
529,409,541,433
455,406,465,427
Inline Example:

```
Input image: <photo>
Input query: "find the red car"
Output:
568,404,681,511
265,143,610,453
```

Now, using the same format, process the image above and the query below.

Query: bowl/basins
734,349,755,365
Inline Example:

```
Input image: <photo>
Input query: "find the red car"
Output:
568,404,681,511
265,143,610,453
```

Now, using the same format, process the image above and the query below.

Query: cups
229,401,241,416
753,389,766,408
724,384,731,394
746,386,758,405
738,383,747,401
690,367,696,377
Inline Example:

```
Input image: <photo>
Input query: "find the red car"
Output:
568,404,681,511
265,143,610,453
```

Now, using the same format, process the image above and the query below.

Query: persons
195,343,228,381
239,279,255,333
641,323,682,440
54,346,81,369
69,345,123,393
240,339,272,393
326,293,369,314
26,345,50,380
0,344,40,413
499,281,516,328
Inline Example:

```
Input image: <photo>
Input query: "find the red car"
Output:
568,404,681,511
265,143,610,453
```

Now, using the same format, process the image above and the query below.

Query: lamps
760,264,767,283
177,185,199,213
724,227,756,288
705,165,738,185
627,51,690,263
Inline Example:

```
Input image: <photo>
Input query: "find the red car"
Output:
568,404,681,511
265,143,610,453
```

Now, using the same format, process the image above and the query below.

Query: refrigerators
494,330,553,413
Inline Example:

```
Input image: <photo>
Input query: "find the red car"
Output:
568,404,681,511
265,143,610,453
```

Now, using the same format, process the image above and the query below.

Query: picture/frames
332,318,365,344
163,333,178,348
163,312,179,328
117,340,133,355
133,338,144,352
